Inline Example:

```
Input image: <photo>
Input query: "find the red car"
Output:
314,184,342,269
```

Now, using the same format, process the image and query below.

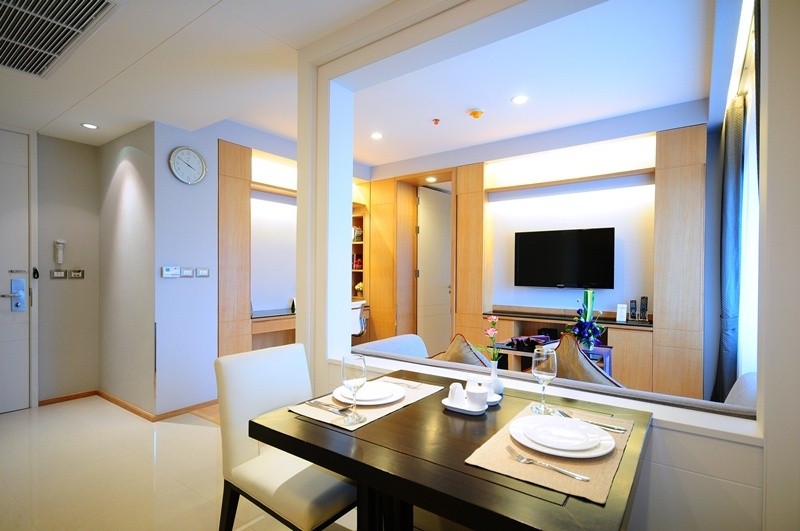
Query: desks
248,369,653,531
487,340,612,379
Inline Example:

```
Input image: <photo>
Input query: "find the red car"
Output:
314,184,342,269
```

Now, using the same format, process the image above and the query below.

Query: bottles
466,373,494,400
448,382,466,406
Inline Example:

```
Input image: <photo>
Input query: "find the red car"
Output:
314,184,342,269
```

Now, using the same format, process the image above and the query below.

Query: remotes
640,296,648,319
629,299,636,319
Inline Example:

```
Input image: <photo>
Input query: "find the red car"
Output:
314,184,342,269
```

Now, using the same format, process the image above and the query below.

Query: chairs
214,343,358,531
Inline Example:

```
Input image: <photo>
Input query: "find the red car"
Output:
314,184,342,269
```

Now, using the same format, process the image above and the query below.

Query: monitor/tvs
514,227,615,290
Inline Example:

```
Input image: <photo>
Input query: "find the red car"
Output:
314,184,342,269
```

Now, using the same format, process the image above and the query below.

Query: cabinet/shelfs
352,207,369,306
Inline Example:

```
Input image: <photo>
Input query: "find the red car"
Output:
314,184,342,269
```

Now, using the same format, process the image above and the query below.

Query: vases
489,361,503,396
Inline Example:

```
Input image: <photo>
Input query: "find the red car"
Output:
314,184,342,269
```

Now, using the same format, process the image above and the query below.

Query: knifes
306,401,349,418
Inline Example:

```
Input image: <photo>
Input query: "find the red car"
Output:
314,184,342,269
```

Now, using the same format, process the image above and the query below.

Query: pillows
425,333,491,367
523,333,626,389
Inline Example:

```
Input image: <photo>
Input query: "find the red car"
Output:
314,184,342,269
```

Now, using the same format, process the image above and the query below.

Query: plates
439,397,488,415
487,391,502,405
342,384,392,401
523,415,601,451
509,416,616,459
333,381,406,405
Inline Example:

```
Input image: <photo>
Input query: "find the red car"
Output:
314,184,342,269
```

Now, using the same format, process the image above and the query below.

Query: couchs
352,335,757,419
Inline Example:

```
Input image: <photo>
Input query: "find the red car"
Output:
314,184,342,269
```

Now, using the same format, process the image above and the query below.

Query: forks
504,445,591,481
384,379,423,388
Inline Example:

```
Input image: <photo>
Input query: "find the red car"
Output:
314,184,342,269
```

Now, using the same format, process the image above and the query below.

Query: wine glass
339,354,367,425
531,348,558,415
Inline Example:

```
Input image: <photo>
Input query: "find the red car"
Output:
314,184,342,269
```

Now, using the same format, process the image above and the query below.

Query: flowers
484,315,502,361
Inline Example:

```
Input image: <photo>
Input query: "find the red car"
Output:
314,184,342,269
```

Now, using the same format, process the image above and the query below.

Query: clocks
169,146,208,185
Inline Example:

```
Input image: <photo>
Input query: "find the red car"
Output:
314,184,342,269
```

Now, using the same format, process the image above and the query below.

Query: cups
463,387,488,409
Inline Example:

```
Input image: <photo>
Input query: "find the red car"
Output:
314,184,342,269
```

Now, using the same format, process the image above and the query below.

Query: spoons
556,409,627,431
314,400,353,412
